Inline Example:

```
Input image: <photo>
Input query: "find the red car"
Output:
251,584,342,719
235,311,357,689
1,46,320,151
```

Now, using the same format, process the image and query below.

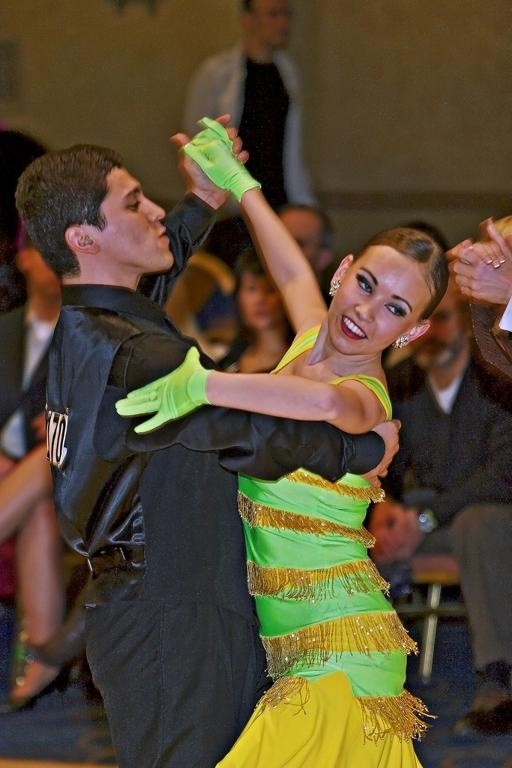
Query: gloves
183,115,262,201
114,347,214,434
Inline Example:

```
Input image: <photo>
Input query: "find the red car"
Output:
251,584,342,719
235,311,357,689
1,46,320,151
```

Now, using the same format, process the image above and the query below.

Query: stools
394,552,466,678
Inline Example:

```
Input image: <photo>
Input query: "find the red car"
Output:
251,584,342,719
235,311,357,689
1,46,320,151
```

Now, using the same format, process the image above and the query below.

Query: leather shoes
12,646,70,704
453,683,511,739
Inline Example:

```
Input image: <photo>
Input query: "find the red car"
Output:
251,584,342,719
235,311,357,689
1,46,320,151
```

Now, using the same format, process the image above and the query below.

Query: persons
180,1,315,251
1,115,512,768
